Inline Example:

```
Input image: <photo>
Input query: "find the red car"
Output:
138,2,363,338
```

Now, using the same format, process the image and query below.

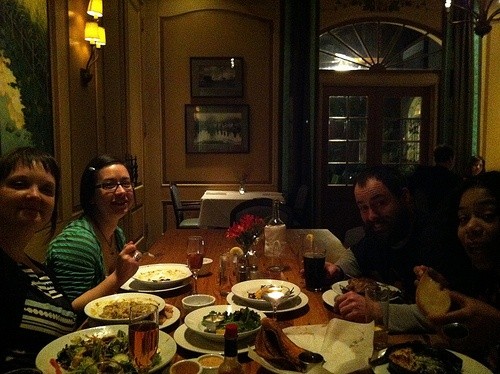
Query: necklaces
93,217,115,256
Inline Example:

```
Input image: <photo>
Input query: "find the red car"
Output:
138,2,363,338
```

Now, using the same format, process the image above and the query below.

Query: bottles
238,198,285,282
218,323,244,374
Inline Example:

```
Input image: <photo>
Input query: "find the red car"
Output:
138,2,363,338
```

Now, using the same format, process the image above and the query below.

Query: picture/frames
183,102,252,155
188,54,246,99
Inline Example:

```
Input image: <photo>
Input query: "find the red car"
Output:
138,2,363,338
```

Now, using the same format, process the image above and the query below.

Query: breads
415,271,452,317
255,316,324,373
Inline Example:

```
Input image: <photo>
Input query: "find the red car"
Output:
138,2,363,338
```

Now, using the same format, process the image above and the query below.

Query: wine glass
261,264,291,318
187,235,206,295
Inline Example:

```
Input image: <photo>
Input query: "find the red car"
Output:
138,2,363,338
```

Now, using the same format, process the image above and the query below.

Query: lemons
230,247,244,256
307,234,313,242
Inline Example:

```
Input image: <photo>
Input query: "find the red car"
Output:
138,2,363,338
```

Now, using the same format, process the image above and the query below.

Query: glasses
94,179,134,192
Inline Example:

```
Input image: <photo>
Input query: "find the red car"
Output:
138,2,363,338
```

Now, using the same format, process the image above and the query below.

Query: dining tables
67,220,393,374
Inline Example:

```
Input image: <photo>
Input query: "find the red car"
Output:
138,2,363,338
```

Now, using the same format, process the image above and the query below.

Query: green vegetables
54,328,162,374
201,305,261,334
377,288,400,301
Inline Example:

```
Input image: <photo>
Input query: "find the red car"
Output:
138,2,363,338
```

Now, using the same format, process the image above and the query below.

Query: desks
195,188,282,227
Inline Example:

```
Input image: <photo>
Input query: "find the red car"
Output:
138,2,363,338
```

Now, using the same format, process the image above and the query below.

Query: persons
46,154,143,322
0,146,79,374
300,144,500,364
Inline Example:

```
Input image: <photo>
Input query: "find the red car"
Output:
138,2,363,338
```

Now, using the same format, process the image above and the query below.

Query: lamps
79,0,106,88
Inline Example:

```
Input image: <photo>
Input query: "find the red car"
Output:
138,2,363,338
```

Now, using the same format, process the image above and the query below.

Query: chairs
168,182,200,229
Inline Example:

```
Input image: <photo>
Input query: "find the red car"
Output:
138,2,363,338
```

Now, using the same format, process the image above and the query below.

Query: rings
135,253,141,260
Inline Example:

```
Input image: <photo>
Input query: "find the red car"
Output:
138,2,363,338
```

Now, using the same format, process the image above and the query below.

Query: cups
219,247,326,295
129,304,225,374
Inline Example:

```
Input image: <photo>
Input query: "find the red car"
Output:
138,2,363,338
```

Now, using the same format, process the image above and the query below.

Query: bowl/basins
184,306,267,341
386,342,463,374
332,280,402,301
230,278,302,305
132,264,192,285
182,258,216,312
84,292,166,322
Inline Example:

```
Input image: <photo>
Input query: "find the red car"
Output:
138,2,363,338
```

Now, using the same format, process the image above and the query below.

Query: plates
322,289,339,306
158,303,180,328
226,292,309,313
373,348,494,374
36,324,178,374
174,323,257,354
121,277,192,291
